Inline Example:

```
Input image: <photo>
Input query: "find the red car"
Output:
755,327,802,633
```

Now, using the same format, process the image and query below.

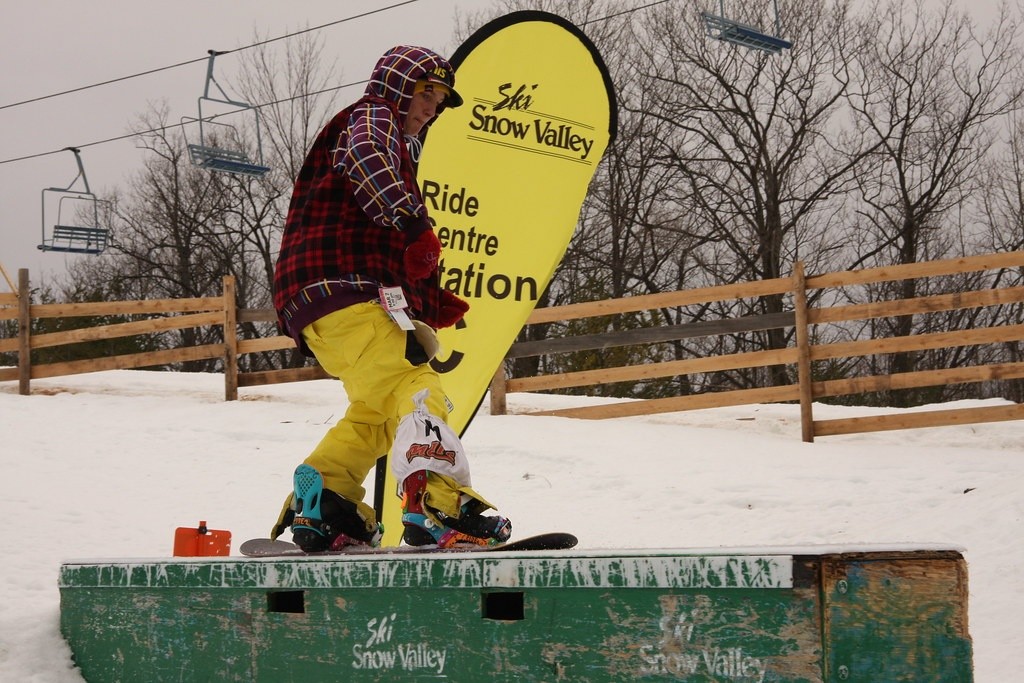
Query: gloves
424,290,471,330
404,230,441,279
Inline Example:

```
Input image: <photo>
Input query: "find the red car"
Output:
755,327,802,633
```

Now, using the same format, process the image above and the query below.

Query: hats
413,66,463,107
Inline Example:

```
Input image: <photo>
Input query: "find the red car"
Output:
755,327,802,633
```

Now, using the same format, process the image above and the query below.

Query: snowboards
238,532,580,559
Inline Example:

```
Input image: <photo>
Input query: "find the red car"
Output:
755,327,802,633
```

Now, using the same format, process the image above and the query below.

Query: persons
275,45,511,550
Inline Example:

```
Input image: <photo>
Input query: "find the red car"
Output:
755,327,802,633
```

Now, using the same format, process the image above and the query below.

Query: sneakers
404,491,512,547
292,488,384,552
